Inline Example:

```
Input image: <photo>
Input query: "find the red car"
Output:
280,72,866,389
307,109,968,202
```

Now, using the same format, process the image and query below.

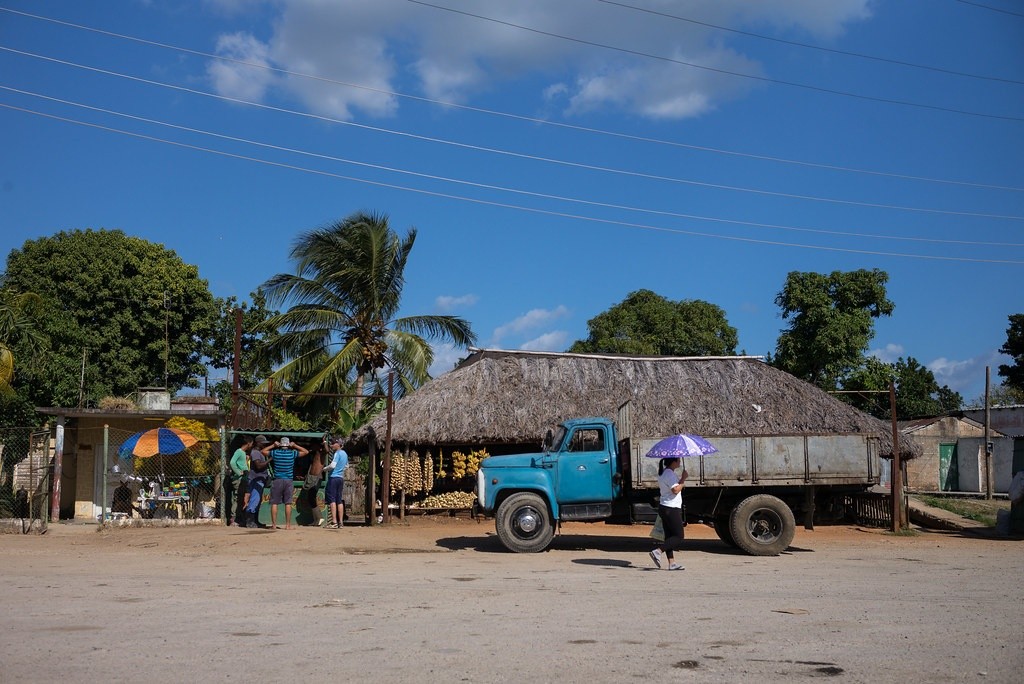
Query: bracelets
322,441,326,444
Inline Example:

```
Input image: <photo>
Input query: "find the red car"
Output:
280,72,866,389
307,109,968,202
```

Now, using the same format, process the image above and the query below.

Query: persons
261,437,309,529
230,436,253,528
323,440,350,529
649,458,689,570
113,477,138,516
302,434,330,527
246,435,272,528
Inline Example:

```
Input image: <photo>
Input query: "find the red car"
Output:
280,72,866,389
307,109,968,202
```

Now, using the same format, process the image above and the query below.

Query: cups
159,482,188,496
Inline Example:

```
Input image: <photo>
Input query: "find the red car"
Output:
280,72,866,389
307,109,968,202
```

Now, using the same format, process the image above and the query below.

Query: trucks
471,400,883,553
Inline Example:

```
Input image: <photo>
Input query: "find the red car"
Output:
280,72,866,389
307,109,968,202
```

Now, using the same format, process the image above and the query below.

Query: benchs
98,512,129,520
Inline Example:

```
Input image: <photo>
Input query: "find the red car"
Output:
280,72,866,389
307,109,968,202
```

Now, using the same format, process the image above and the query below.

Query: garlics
418,490,478,509
391,450,433,496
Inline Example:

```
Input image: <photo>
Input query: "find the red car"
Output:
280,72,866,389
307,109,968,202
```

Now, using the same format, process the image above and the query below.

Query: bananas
452,449,490,478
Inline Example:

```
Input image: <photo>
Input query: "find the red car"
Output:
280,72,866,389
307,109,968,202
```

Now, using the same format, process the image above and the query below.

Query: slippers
669,566,685,570
649,552,661,569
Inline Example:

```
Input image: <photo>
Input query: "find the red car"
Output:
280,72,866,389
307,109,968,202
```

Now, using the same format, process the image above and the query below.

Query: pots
119,471,143,484
112,465,121,473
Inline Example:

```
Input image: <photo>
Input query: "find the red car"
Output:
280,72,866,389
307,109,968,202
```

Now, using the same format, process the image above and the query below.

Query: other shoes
230,518,240,527
324,522,343,529
246,523,266,528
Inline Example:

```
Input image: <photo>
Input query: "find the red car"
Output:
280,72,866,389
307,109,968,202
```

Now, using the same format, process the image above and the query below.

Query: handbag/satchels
649,514,665,542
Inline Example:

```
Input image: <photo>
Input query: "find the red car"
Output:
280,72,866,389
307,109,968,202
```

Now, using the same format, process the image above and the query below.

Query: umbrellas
646,434,717,472
116,427,198,474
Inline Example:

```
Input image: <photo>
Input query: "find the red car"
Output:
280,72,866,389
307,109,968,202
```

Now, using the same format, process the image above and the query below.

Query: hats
255,435,269,443
331,436,343,446
279,437,290,446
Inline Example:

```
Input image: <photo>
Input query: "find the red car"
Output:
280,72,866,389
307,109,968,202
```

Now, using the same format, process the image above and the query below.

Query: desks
137,494,191,519
